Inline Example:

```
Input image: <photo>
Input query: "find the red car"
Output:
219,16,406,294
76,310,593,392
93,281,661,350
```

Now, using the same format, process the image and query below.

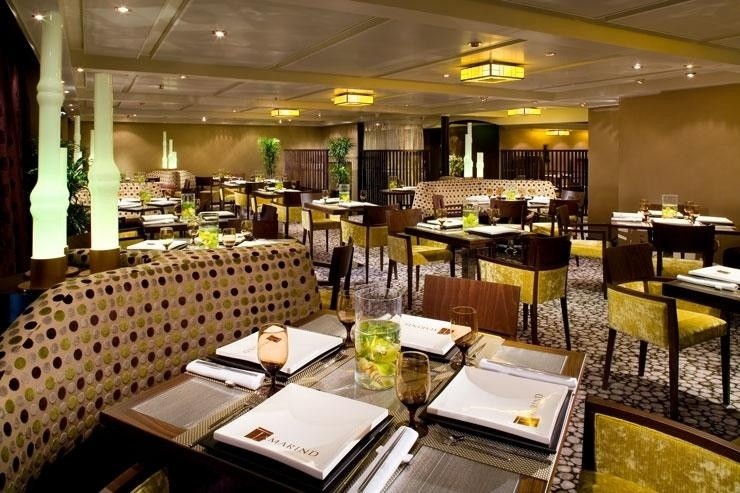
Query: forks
188,391,266,448
425,424,555,468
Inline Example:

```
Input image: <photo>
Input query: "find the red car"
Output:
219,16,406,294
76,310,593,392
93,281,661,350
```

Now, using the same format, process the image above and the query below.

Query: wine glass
395,351,431,439
449,305,478,372
336,288,364,349
258,323,288,397
121,171,700,253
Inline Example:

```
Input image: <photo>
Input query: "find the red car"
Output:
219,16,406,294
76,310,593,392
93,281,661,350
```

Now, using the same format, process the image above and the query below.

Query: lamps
544,129,570,136
458,59,526,83
333,89,374,106
507,107,541,116
271,108,300,118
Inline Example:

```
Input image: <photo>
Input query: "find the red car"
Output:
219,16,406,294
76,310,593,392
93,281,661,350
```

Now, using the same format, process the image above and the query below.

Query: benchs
1,239,322,493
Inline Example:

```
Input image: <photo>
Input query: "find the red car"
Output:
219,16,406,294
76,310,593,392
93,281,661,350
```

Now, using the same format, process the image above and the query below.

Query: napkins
675,273,738,292
479,357,579,389
348,425,420,493
186,358,263,390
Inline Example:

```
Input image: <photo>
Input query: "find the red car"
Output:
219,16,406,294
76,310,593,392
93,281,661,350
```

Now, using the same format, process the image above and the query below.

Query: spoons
313,348,348,378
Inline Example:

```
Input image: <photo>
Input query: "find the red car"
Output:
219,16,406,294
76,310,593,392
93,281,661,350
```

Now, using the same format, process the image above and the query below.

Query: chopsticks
333,424,408,493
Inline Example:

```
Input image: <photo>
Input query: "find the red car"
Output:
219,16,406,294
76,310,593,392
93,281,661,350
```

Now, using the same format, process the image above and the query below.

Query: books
385,312,472,364
417,362,575,455
118,194,275,252
689,262,740,283
312,193,552,238
190,382,397,492
611,208,738,230
208,320,348,382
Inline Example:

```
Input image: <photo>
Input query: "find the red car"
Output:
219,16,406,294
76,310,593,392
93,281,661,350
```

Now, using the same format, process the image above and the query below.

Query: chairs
299,172,586,349
601,243,729,420
76,170,326,253
577,394,740,491
653,222,715,280
570,204,738,265
